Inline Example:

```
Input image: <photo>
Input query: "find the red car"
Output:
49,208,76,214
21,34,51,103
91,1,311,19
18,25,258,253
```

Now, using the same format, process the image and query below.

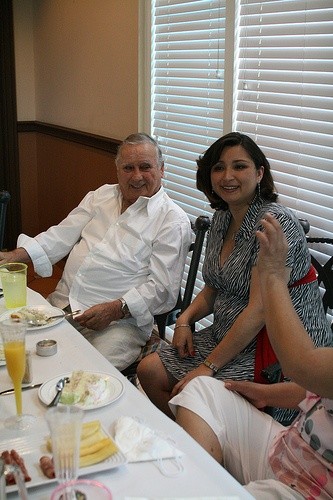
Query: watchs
118,298,130,319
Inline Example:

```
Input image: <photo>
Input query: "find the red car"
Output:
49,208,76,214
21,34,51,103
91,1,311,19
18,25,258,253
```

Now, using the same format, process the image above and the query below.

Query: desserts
10,308,48,324
59,370,106,406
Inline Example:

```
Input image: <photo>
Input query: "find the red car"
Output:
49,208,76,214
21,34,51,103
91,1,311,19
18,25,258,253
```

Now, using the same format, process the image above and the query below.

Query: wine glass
0,318,37,431
46,406,84,499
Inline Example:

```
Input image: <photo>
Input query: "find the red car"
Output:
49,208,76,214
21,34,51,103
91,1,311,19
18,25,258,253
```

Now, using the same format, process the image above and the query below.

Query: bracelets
174,324,191,330
203,359,219,373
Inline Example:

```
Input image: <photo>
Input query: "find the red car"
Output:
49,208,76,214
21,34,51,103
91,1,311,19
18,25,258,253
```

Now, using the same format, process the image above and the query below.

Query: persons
137,131,333,427
168,213,333,500
0,133,192,372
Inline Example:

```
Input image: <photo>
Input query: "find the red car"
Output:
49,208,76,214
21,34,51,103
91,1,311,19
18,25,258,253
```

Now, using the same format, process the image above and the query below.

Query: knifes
0,384,42,395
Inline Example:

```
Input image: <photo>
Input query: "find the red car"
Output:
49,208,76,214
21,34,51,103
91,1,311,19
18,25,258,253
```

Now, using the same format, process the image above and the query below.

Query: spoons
28,310,80,326
47,377,70,408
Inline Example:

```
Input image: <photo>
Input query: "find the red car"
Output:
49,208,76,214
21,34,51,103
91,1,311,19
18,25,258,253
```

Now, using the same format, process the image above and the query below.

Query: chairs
121,215,210,376
299,218,333,315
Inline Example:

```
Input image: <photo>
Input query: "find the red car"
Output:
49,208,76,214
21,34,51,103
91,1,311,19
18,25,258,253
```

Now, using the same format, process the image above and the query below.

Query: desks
0,268,255,500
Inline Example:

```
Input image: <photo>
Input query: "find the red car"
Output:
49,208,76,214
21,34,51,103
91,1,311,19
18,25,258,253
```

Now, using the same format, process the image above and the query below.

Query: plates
0,419,127,494
37,371,125,409
0,304,65,330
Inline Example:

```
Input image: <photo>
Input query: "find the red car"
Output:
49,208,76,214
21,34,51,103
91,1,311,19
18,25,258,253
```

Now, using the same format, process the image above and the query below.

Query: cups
22,349,33,384
36,339,57,356
0,262,28,309
50,480,112,500
